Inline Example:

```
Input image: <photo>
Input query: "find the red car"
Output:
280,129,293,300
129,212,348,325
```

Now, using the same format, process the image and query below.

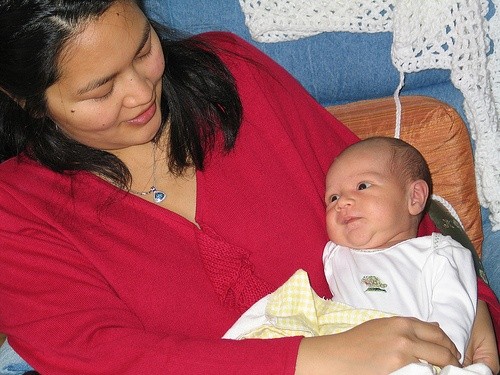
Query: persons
0,0,500,375
322,135,478,364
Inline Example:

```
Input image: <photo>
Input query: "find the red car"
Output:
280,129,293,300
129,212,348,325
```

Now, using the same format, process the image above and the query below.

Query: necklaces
90,136,167,203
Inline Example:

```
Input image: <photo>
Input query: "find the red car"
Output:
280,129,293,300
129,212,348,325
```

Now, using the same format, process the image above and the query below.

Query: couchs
0,0,500,375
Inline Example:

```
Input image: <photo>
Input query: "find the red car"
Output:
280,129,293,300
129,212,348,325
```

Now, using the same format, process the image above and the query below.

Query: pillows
325,96,483,257
